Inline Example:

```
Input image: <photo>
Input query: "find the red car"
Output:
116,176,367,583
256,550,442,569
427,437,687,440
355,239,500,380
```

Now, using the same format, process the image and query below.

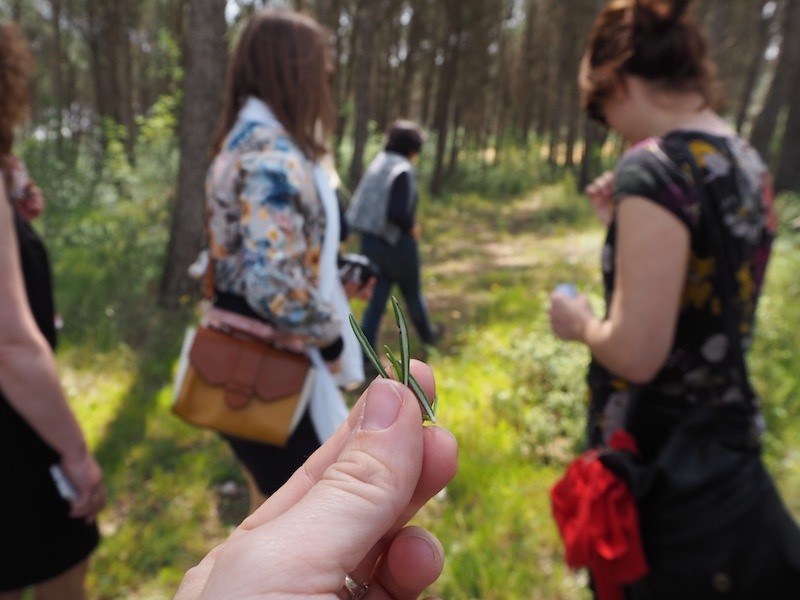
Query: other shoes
422,320,445,348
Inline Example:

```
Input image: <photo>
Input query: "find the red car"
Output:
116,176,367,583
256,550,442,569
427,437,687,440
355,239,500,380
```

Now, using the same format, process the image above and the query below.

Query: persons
206,11,363,519
342,119,446,365
0,20,107,600
551,0,800,600
172,360,458,600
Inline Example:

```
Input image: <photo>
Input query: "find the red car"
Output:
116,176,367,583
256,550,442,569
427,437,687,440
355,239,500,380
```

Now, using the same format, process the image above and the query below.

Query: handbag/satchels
170,308,317,446
546,452,648,599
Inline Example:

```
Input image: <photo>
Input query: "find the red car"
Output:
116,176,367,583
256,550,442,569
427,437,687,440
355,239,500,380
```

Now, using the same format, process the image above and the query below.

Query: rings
344,574,372,600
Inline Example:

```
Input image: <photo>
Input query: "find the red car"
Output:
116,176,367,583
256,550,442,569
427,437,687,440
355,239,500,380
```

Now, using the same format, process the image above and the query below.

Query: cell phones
49,464,78,502
